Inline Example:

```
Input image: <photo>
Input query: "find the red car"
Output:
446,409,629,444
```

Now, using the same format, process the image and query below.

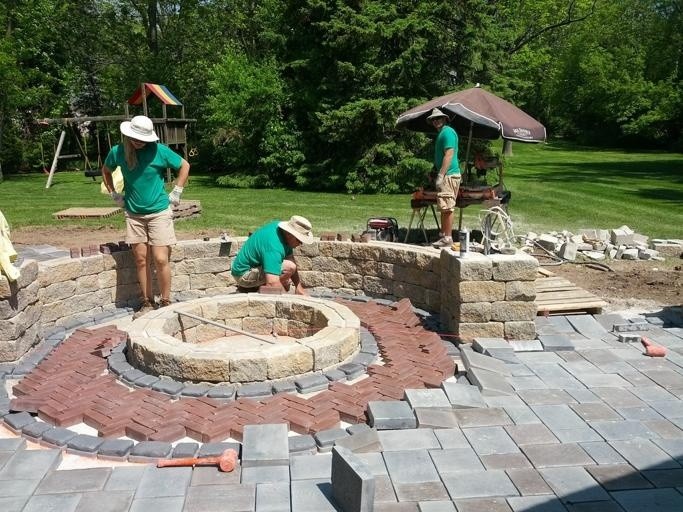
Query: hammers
156,448,237,471
641,335,666,356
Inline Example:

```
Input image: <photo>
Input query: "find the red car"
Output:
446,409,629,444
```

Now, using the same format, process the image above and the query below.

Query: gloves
110,191,125,207
436,173,444,189
169,187,183,204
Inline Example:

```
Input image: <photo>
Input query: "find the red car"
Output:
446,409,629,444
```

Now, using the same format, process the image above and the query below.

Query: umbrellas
394,82,547,232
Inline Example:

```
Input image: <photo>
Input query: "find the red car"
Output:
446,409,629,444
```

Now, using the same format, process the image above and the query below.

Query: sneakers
133,298,170,319
432,237,454,246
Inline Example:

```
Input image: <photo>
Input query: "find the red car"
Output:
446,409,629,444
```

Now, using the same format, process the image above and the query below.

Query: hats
121,114,159,142
278,215,313,245
427,108,450,124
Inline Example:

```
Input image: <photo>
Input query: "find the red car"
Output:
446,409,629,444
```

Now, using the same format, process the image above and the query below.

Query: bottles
459,226,470,259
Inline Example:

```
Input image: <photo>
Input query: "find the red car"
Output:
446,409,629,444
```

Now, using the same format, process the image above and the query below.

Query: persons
231,216,314,296
426,107,462,247
101,115,191,320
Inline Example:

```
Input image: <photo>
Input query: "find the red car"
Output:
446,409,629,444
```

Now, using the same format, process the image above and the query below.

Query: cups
7,254,24,267
